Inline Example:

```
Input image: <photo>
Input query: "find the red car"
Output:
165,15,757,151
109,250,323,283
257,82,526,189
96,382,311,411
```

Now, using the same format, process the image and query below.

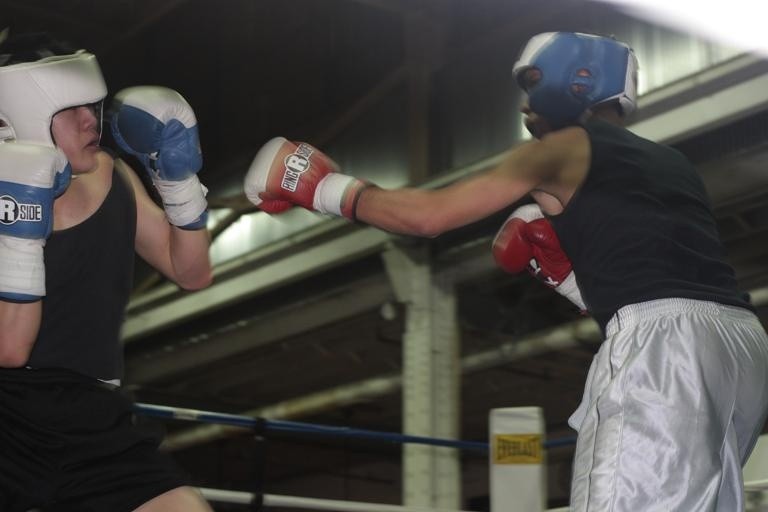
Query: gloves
0,138,72,305
110,83,212,230
244,134,371,230
490,202,592,316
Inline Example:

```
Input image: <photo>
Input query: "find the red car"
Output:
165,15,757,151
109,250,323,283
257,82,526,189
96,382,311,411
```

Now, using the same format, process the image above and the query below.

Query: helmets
510,29,640,143
1,50,107,196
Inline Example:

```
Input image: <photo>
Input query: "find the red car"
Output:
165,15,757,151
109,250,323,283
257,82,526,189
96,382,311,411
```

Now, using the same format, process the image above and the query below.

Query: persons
242,33,768,512
0,30,218,512
